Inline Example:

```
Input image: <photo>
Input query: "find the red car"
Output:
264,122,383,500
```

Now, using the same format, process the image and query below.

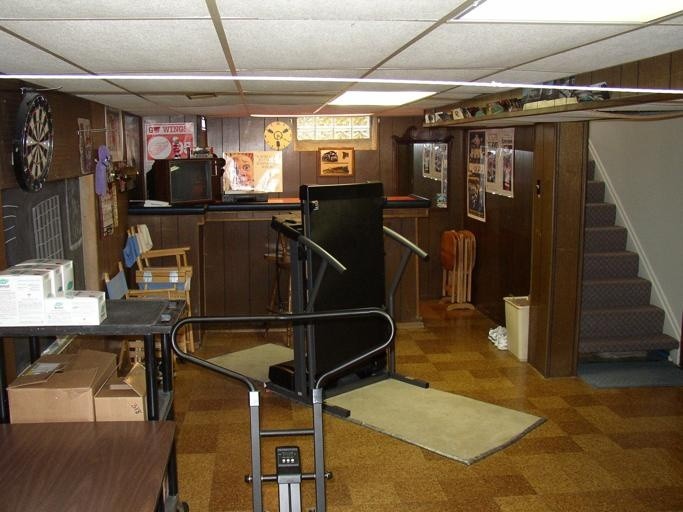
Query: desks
0,421,177,512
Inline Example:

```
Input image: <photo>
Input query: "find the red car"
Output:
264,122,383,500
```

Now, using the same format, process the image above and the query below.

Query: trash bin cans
503,296,530,362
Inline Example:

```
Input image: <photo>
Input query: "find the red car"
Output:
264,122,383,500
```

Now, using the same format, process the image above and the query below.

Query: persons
469,133,512,213
225,154,254,190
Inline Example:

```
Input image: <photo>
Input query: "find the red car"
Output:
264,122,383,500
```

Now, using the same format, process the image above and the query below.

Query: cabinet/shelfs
0,298,187,512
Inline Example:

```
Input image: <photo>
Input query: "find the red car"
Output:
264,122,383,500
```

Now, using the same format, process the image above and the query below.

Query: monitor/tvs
145,158,213,205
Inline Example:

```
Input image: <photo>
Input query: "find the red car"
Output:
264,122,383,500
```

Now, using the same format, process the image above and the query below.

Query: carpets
206,343,546,465
578,359,682,388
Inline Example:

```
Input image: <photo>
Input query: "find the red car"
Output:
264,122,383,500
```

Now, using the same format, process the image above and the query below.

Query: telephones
144,200,172,208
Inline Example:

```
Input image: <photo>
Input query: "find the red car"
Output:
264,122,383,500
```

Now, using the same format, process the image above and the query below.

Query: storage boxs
94,361,148,421
7,349,117,424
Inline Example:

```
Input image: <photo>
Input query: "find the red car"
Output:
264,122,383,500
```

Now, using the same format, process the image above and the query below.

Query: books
128,199,171,208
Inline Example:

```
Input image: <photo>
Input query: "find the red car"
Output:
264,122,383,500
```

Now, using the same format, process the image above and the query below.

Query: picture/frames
318,147,355,179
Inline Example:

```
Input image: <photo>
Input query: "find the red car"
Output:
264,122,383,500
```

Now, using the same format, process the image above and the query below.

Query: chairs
104,223,195,380
264,232,293,347
